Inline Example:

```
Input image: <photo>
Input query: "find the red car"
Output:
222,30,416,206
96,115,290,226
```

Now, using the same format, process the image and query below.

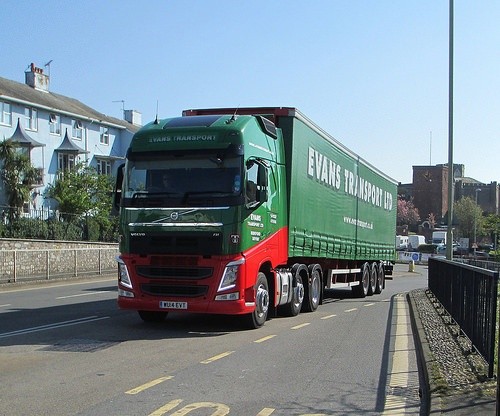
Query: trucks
396,235,425,251
432,232,447,247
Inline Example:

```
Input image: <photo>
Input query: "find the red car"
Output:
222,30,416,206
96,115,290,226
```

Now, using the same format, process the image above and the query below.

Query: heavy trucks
114,108,398,328
